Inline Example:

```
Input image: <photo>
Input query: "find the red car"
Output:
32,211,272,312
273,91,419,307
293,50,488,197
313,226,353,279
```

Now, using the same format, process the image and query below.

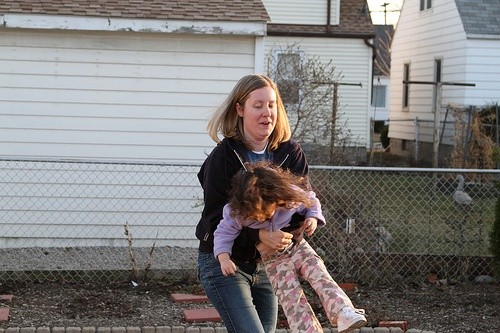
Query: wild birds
452,175,473,205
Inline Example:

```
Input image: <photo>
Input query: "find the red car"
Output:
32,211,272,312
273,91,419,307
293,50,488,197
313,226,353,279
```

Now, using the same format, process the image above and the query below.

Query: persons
195,74,310,333
213,161,368,333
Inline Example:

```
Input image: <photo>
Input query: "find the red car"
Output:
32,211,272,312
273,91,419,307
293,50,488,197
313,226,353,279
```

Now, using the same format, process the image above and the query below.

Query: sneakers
337,306,368,333
